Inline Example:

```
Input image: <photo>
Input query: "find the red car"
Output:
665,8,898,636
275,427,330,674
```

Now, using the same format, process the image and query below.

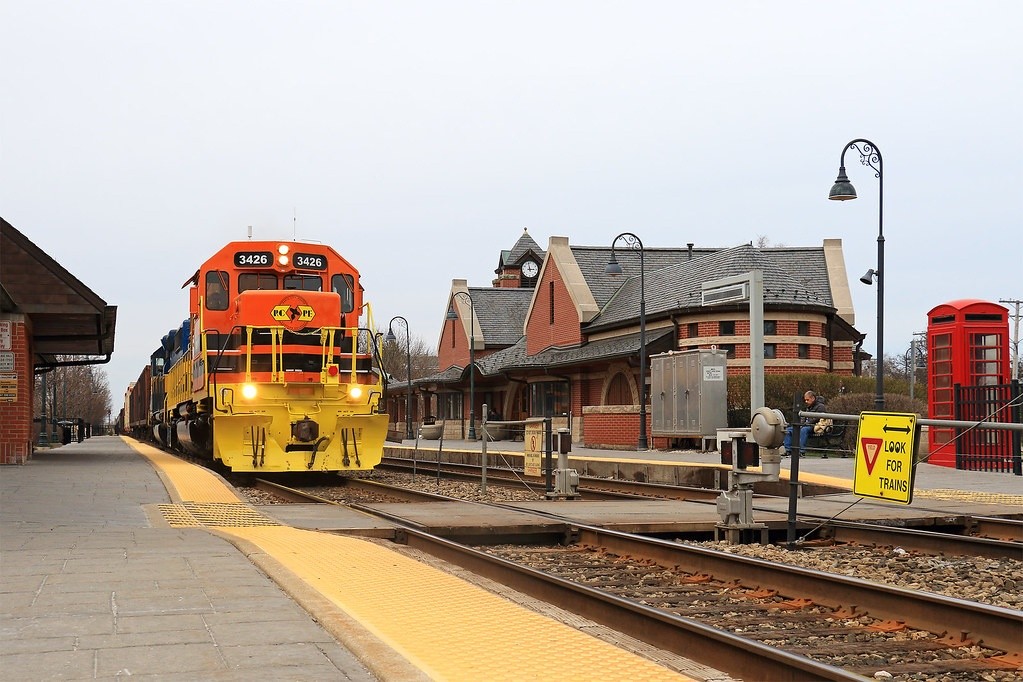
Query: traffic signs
853,410,921,505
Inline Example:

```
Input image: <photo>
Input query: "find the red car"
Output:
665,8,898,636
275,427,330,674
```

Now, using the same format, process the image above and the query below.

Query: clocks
522,260,538,278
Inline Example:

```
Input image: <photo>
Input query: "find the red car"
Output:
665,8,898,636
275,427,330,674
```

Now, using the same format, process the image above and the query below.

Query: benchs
509,419,526,443
806,419,849,459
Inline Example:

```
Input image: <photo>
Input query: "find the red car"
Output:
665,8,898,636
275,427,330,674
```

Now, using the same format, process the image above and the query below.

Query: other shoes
780,449,791,456
799,452,806,458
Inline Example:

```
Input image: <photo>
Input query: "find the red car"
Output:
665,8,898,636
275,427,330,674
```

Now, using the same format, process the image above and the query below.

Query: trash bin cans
58,421,74,446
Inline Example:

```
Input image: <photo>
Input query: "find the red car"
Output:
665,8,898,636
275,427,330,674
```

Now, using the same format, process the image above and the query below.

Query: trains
115,209,391,485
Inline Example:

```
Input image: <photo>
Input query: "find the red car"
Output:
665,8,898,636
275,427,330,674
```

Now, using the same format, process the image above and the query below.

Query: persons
783,390,828,458
488,408,502,421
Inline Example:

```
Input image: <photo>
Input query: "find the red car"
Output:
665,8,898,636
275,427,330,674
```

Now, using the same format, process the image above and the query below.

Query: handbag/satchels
814,418,834,433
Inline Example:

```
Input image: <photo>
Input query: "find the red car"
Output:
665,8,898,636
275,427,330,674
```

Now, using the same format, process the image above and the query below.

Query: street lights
386,316,414,440
828,138,886,411
444,292,478,442
604,231,649,452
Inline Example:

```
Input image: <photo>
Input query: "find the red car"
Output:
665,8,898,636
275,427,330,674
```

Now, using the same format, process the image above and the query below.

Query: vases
422,424,443,439
482,424,505,442
